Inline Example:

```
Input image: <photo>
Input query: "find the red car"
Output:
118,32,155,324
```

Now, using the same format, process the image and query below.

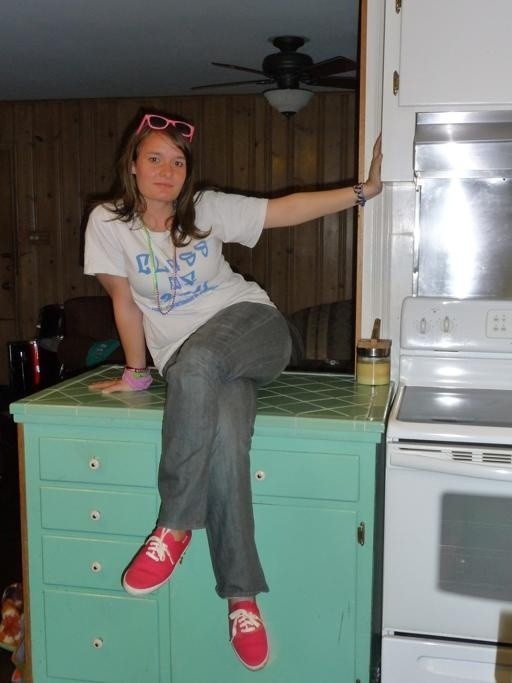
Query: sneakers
228,597,269,671
122,526,192,598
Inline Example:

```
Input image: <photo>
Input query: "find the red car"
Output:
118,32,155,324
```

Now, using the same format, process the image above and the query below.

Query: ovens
382,441,511,681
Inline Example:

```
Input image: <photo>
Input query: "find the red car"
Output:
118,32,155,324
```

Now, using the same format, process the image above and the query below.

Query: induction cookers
397,385,512,426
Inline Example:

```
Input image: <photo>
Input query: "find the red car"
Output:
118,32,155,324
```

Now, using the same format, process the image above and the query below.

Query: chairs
58,295,126,374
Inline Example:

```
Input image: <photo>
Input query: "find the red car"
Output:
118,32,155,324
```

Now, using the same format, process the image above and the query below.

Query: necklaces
144,226,176,315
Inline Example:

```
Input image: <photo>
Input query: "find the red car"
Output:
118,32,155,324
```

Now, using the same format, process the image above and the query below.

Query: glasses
134,113,197,143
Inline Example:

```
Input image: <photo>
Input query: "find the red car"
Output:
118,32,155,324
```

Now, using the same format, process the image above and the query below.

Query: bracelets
353,184,367,207
122,365,153,391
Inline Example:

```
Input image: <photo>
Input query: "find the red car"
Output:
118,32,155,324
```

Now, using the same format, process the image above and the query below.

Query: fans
190,34,358,94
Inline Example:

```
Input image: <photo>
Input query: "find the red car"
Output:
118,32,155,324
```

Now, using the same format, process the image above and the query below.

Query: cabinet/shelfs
7,365,397,683
381,2,511,189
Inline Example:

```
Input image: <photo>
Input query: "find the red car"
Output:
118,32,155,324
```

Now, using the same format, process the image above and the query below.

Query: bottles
356,339,390,385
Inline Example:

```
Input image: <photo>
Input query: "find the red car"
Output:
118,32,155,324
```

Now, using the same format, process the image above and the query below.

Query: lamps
259,82,317,119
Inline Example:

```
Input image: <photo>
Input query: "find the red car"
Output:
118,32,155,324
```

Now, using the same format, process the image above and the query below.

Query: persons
83,113,382,671
